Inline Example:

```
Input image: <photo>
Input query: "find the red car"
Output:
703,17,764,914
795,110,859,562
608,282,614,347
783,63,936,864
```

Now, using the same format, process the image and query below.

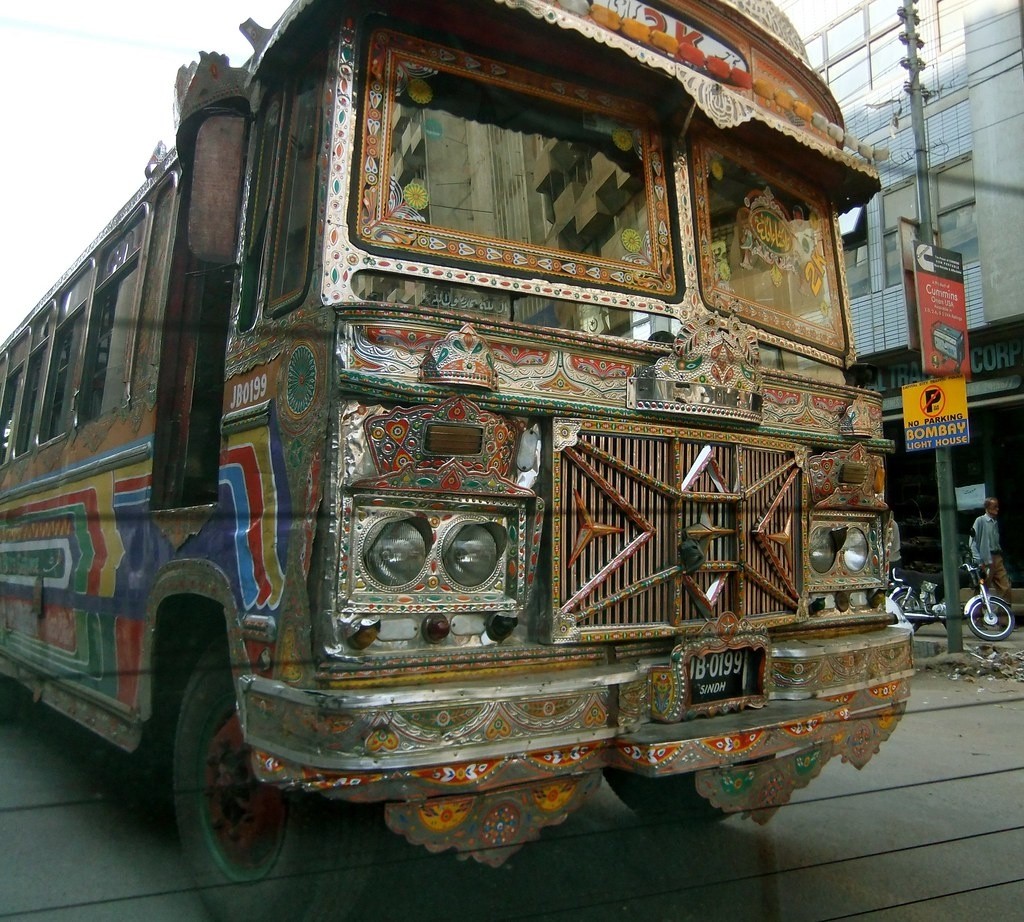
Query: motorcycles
887,527,1016,642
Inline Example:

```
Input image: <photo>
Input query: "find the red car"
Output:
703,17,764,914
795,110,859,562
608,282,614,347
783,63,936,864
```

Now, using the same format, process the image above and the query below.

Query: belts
990,551,1001,554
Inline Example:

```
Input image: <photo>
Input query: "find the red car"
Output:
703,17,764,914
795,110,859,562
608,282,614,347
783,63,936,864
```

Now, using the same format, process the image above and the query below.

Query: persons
969,497,1018,629
889,511,902,578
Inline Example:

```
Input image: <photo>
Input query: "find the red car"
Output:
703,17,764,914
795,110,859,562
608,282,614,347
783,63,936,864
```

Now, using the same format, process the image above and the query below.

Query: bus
0,0,919,922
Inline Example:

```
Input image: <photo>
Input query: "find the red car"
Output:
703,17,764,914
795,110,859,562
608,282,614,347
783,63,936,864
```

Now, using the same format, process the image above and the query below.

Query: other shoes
999,623,1018,633
974,620,988,631
